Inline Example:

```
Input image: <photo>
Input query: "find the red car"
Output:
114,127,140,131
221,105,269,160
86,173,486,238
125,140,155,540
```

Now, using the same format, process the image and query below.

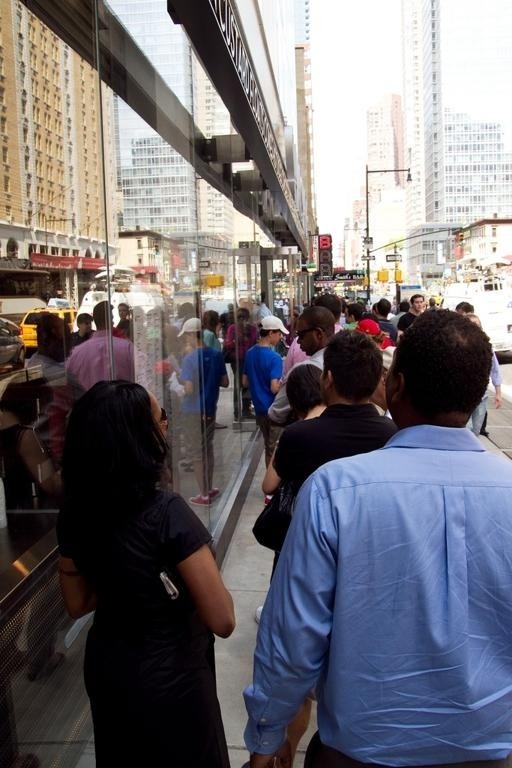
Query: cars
174,287,192,295
0,318,26,369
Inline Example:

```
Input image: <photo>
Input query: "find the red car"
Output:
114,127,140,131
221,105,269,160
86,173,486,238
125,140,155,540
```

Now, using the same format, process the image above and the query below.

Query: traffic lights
155,245,160,255
458,229,464,242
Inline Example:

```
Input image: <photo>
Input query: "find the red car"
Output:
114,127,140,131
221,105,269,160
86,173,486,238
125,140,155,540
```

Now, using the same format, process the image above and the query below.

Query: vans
73,286,167,344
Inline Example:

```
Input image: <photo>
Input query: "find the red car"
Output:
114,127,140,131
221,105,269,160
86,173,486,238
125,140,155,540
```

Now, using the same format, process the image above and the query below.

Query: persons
0,475,44,768
242,291,502,508
239,308,510,766
57,376,238,765
2,300,242,679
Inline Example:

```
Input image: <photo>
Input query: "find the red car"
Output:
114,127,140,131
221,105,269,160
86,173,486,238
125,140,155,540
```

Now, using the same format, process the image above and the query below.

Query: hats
259,315,290,335
177,318,200,338
356,319,381,335
382,346,396,370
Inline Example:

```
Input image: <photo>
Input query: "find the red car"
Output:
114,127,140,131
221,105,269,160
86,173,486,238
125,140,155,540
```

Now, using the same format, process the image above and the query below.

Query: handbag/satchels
252,480,296,552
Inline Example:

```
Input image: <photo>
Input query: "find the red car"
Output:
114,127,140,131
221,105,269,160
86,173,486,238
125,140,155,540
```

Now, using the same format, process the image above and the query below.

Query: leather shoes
214,422,227,429
27,651,66,681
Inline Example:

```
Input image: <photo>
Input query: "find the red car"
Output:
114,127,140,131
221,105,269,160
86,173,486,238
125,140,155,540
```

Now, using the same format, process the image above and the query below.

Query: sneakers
255,606,263,625
264,494,273,506
209,488,220,499
189,496,212,506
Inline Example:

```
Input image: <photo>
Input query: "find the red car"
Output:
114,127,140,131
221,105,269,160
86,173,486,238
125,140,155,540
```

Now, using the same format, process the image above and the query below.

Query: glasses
295,328,312,340
158,408,168,422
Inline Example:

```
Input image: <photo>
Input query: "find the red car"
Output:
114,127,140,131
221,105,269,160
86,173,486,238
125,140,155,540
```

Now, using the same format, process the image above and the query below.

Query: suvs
18,298,80,350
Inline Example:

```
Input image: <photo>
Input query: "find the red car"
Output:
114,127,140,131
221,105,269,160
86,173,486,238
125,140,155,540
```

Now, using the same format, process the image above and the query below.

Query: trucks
440,280,512,352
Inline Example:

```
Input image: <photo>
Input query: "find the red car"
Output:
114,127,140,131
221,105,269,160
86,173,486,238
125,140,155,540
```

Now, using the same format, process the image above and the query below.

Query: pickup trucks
399,284,430,304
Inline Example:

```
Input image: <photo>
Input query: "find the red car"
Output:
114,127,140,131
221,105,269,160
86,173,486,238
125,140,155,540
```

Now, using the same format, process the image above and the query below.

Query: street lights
365,165,412,302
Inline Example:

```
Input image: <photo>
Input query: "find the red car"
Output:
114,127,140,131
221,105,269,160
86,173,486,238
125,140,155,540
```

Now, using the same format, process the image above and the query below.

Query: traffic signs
198,261,209,268
362,256,375,260
385,254,402,262
